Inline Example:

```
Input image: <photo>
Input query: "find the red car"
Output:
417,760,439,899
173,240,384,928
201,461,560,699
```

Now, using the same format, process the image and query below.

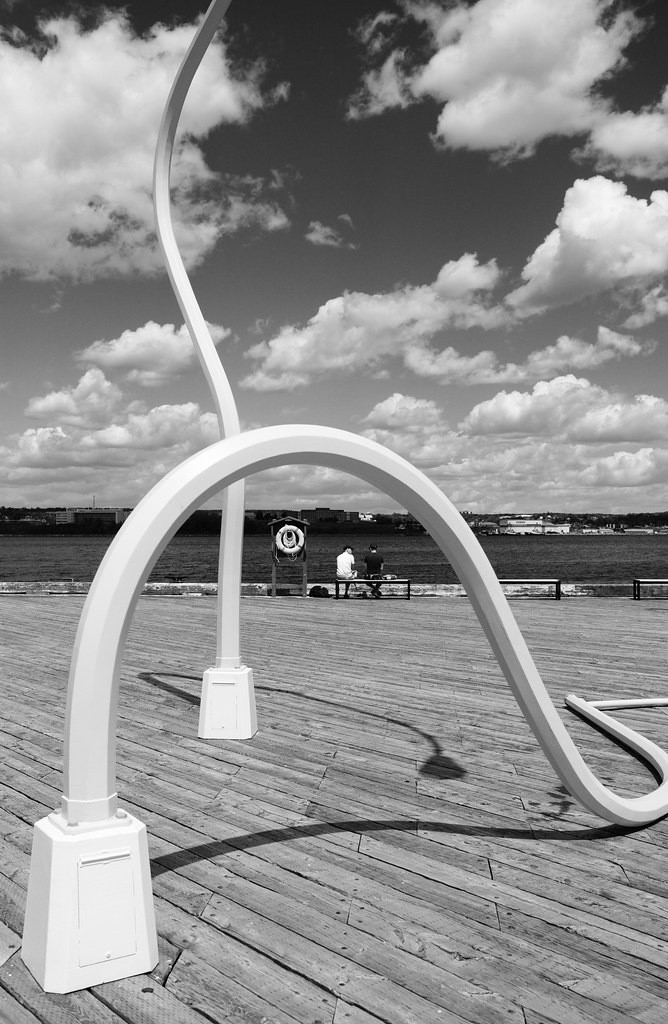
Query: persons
336,545,358,599
364,543,384,597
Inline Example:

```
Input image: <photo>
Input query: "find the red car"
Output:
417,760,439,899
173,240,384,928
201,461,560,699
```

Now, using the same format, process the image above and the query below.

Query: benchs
498,579,561,600
336,579,410,600
633,579,668,600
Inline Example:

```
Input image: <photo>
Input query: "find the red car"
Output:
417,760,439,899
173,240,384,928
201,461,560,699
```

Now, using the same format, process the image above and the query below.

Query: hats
343,545,354,550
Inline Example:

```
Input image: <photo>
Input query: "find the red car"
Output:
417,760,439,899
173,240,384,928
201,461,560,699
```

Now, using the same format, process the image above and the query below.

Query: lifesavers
275,525,305,553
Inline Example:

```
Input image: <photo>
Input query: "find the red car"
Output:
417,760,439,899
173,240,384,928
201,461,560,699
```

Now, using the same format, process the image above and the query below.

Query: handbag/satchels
309,586,332,598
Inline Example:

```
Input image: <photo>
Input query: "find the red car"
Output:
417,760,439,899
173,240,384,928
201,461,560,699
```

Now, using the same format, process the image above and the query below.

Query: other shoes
376,590,382,596
372,593,380,598
